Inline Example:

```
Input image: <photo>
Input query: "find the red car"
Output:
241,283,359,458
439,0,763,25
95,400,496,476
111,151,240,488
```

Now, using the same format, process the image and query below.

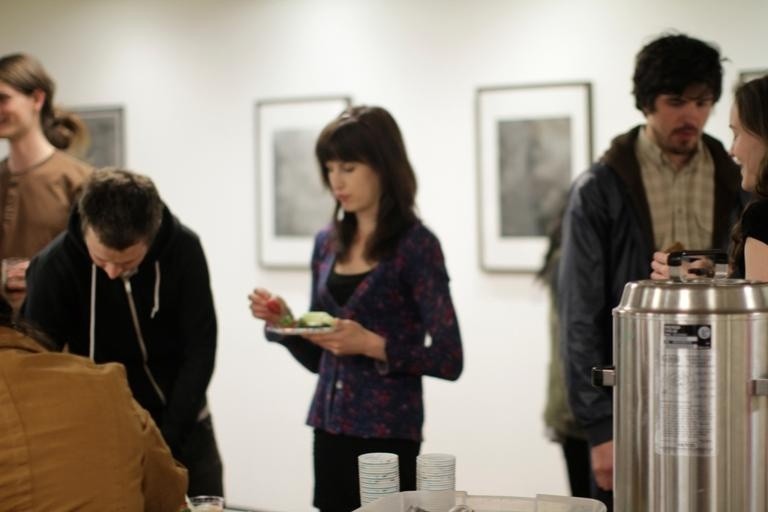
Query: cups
357,452,400,506
185,495,225,512
416,453,456,491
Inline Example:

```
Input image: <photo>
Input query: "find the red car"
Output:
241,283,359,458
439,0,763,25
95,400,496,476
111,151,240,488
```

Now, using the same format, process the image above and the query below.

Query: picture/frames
252,96,352,273
53,105,126,171
475,81,593,274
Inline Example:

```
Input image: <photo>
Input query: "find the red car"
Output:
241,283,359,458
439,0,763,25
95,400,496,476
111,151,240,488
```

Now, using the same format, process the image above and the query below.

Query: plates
264,324,337,337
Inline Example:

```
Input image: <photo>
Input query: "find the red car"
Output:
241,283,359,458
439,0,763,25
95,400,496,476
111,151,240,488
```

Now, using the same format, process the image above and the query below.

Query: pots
591,249,768,512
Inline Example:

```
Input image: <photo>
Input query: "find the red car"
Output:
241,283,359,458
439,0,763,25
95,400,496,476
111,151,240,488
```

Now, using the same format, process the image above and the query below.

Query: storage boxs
350,488,608,512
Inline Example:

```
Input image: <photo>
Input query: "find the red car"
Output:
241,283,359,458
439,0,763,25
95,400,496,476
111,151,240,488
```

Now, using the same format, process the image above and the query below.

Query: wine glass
0,255,31,323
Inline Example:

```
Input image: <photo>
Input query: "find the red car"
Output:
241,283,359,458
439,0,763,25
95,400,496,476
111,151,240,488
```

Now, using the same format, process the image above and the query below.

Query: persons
533,169,592,496
649,69,768,283
248,101,463,511
557,31,739,511
0,294,194,511
13,164,225,502
0,53,100,322
